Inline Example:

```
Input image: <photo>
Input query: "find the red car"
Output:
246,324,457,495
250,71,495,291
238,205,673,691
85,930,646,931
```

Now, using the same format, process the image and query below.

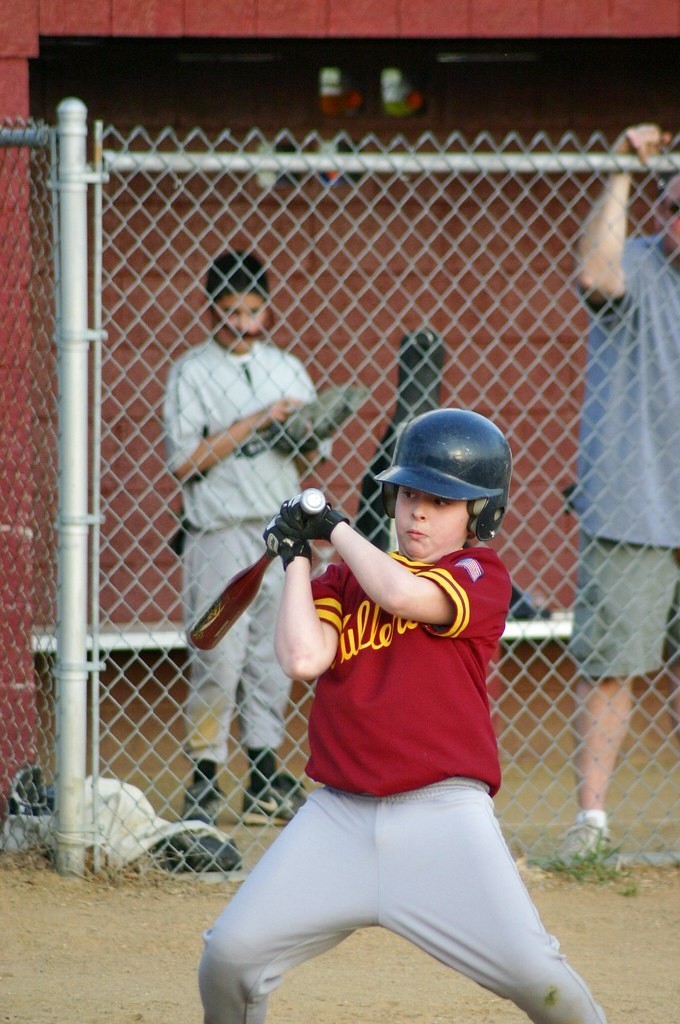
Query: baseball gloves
274,386,367,451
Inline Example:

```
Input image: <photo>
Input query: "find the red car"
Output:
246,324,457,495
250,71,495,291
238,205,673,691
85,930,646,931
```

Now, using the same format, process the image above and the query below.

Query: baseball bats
189,488,326,651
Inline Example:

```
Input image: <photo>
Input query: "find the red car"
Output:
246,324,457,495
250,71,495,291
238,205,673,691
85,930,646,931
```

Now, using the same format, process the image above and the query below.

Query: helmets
204,250,270,302
372,407,515,543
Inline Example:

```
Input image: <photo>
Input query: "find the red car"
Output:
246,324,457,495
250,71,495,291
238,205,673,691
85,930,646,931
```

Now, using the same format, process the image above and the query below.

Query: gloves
262,492,351,572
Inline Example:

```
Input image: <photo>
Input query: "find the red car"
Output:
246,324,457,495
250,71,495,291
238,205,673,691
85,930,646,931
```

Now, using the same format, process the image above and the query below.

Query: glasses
669,196,680,219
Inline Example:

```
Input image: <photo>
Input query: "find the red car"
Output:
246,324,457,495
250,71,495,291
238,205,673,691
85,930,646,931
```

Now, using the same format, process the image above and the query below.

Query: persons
162,252,334,827
197,407,608,1024
558,123,680,863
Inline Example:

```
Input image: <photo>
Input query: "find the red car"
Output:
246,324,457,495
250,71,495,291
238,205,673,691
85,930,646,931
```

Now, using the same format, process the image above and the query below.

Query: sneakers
555,818,612,855
181,778,229,827
241,772,308,826
